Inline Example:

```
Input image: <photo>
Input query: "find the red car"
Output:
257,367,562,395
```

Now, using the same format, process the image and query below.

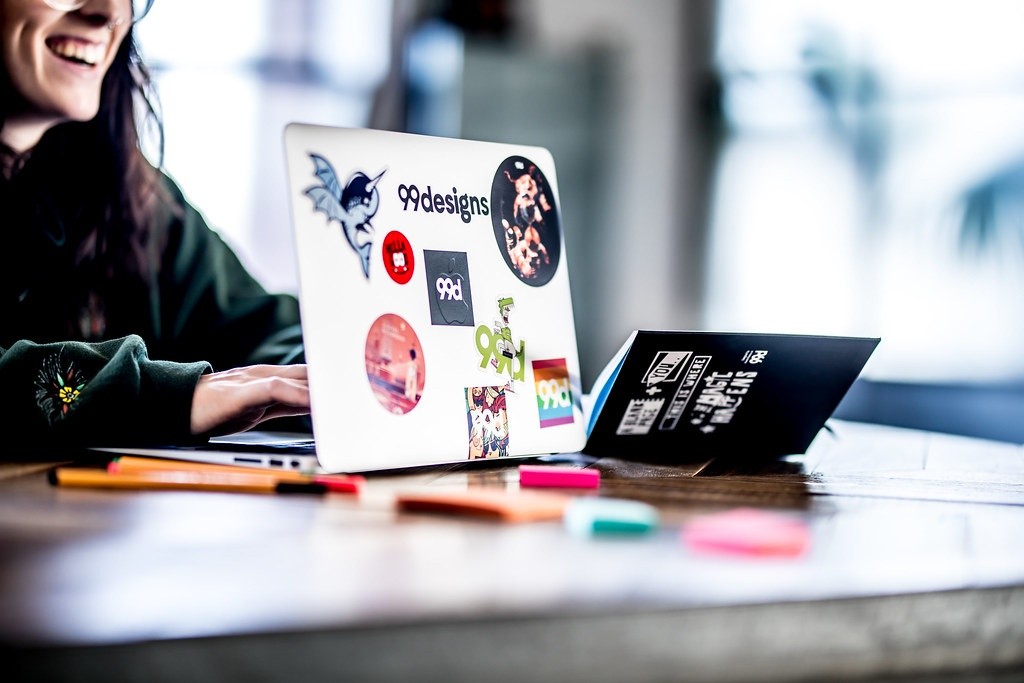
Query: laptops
80,122,587,476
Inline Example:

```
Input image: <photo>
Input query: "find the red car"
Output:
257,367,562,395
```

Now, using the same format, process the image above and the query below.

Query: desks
0,395,1024,683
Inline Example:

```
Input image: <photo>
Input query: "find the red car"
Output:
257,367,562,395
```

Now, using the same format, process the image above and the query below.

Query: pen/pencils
44,465,330,498
108,454,366,491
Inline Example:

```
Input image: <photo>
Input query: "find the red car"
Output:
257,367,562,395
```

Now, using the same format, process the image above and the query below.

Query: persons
0,0,312,452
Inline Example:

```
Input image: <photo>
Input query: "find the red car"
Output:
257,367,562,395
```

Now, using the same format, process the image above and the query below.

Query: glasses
40,0,155,26
